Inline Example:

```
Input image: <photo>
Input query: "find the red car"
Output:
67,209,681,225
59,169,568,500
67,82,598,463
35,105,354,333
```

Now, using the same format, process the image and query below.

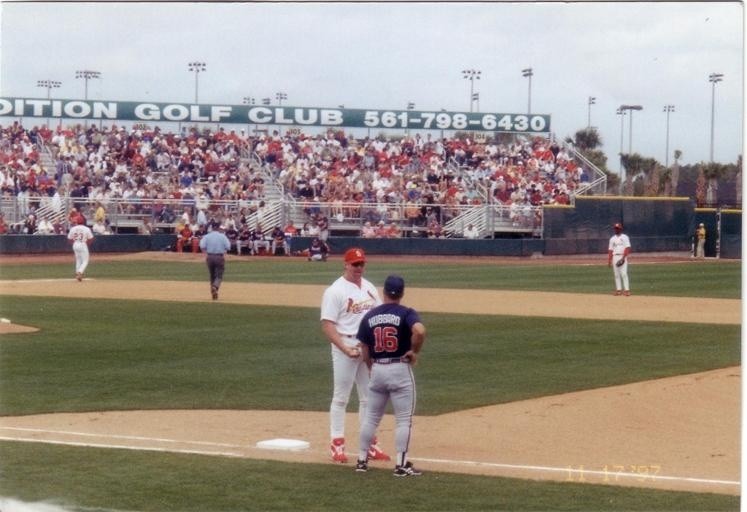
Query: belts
372,358,401,364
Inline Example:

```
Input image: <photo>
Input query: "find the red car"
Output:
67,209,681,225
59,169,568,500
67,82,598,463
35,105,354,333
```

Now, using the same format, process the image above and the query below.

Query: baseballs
353,349,359,353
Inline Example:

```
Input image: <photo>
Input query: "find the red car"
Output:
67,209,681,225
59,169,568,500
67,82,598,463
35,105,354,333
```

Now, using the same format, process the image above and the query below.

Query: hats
384,275,404,294
344,248,368,265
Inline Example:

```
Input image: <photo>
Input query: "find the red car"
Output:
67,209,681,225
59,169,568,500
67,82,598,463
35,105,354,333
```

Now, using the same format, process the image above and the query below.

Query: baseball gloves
616,258,624,267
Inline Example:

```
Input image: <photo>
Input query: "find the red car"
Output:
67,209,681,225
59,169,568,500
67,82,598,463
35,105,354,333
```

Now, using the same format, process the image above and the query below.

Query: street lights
36,77,63,130
707,72,724,163
260,97,272,106
187,61,208,133
275,92,287,137
405,100,415,139
520,64,531,116
583,94,676,189
460,68,483,113
73,69,102,128
241,96,257,138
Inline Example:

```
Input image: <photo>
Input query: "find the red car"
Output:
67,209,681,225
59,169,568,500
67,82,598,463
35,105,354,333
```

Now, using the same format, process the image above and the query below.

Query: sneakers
355,459,368,471
393,461,423,477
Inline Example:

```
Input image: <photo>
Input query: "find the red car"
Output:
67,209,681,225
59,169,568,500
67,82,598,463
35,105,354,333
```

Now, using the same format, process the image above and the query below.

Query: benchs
2,125,580,237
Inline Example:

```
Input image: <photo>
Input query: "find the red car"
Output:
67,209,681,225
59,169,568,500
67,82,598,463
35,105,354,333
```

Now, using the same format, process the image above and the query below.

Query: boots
328,438,348,463
367,437,391,461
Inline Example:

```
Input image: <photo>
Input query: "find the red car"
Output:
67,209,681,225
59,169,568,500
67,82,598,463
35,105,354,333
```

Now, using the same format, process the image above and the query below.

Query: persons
199,219,231,299
67,217,94,281
607,223,632,296
355,274,426,478
695,222,706,259
320,249,391,464
